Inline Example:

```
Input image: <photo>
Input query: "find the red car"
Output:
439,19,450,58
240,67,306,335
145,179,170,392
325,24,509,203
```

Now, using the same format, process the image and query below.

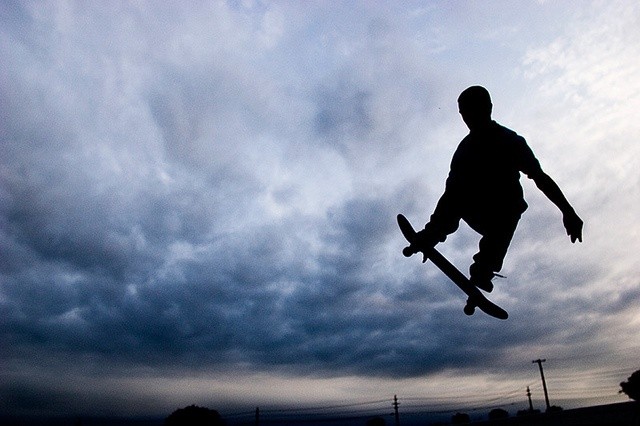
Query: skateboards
396,213,508,319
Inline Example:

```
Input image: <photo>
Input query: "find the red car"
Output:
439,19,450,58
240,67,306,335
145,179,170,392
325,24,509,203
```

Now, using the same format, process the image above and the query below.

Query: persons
416,85,583,292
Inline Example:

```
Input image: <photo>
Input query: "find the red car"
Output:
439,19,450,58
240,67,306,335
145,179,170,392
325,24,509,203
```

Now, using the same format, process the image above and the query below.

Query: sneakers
469,274,506,293
